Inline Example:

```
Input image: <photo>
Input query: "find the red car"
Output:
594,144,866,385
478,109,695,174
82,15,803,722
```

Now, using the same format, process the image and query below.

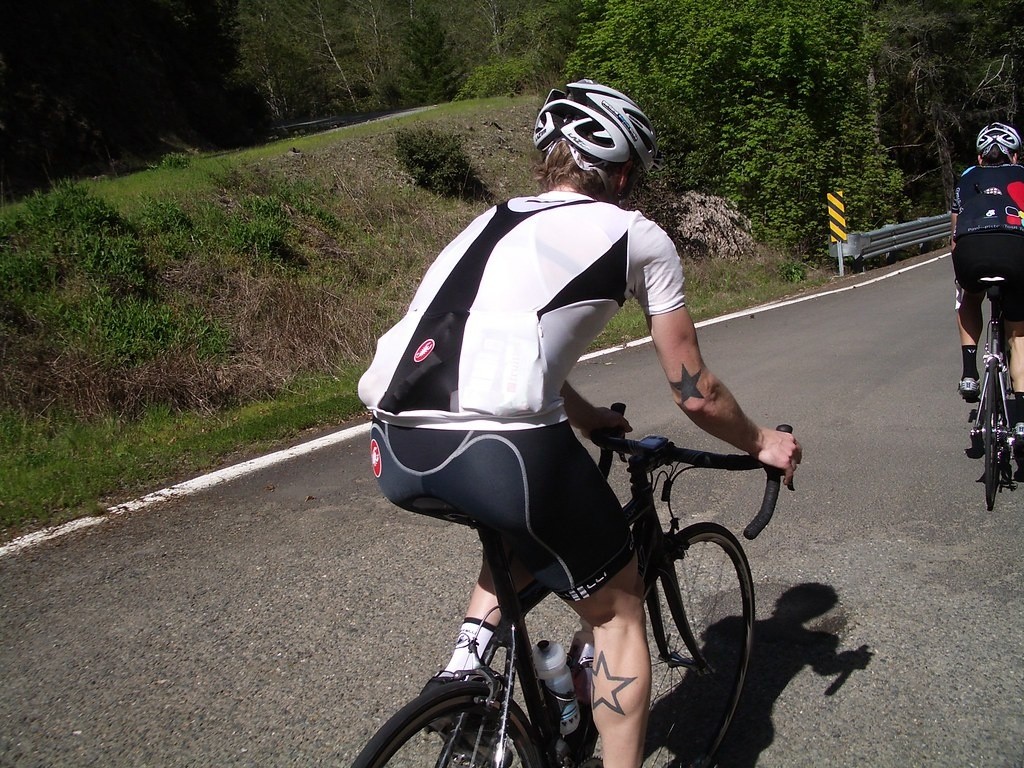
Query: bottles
570,620,597,695
531,639,581,736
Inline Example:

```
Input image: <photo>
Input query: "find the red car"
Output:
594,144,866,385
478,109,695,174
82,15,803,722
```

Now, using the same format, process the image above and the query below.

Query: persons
356,78,801,768
950,122,1024,441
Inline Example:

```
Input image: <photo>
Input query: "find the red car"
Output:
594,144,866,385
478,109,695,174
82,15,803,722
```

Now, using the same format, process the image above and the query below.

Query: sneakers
1014,422,1024,442
419,676,513,768
958,377,980,397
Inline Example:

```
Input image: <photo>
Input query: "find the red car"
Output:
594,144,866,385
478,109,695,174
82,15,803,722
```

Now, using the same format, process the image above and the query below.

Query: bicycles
964,276,1024,512
344,401,798,768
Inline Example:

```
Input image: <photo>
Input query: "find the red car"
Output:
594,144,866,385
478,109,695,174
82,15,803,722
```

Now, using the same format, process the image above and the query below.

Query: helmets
532,79,658,173
976,123,1022,154
